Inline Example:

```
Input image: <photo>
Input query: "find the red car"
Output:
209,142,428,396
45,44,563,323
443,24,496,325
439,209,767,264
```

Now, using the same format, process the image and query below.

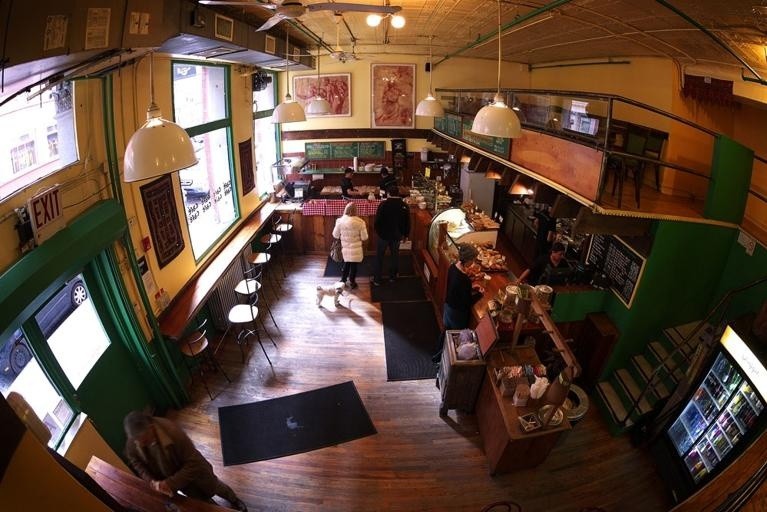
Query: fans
284,15,377,65
199,0,402,33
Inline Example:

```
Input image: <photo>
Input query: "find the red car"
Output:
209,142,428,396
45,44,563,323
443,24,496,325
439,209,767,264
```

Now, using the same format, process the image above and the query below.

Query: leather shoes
341,280,357,289
231,499,248,512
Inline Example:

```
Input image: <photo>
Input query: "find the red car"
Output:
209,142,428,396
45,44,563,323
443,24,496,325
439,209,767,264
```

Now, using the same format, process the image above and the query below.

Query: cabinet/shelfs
487,162,591,282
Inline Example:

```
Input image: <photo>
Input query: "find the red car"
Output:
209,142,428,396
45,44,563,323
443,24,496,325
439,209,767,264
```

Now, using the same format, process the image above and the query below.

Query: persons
341,168,362,199
528,242,569,286
370,185,411,286
331,202,368,290
435,243,485,355
380,168,396,197
124,411,248,512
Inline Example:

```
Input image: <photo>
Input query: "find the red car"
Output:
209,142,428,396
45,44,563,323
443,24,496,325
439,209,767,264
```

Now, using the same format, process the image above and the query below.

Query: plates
358,163,393,172
538,403,564,427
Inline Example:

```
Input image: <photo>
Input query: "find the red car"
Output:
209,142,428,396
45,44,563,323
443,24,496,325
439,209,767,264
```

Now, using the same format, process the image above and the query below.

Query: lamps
415,36,444,117
471,0,522,139
123,53,198,183
305,42,332,115
271,27,306,124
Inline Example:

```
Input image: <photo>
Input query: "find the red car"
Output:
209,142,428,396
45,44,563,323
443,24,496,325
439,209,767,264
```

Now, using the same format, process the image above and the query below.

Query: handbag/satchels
330,239,343,263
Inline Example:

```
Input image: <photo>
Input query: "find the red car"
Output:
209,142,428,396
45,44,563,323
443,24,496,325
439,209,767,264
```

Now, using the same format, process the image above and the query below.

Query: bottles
495,280,554,325
665,352,765,485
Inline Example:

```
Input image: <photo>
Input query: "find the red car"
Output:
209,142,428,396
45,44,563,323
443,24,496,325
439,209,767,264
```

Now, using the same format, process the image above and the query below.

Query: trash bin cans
562,384,589,427
436,310,499,418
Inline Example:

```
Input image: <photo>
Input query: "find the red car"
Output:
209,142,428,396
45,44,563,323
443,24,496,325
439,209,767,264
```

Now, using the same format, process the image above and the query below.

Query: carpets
370,277,427,301
380,301,442,381
324,254,418,277
218,380,377,466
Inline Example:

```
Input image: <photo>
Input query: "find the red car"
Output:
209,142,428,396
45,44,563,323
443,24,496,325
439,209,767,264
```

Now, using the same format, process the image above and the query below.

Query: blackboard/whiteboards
305,141,386,160
585,232,647,310
434,110,511,161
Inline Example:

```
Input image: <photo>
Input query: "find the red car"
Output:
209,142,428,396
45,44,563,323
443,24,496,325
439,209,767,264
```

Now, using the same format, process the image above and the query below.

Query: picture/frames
291,73,351,117
370,62,416,129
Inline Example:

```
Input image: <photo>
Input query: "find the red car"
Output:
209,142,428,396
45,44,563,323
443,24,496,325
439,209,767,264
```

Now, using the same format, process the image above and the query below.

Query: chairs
181,207,292,401
451,95,670,209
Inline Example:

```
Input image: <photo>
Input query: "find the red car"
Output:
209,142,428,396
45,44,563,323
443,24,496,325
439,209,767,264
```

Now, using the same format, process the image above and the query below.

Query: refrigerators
459,167,495,221
646,321,766,503
